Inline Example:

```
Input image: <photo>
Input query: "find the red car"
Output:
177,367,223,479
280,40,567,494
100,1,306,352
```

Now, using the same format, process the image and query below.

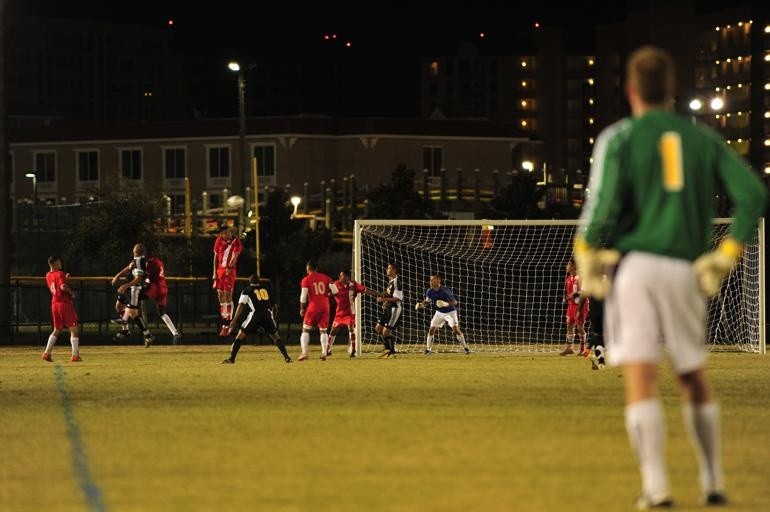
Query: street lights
290,195,302,215
23,171,38,207
224,56,252,238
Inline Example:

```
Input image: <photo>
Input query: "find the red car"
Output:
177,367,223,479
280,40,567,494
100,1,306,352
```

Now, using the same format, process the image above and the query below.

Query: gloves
415,302,424,311
693,251,735,297
574,247,619,300
436,299,449,308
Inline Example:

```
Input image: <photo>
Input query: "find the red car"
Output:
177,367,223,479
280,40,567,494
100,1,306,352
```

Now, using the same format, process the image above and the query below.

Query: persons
576,46,768,509
296,259,340,362
588,296,605,370
557,258,590,357
377,262,405,359
414,273,471,355
212,225,243,336
111,243,155,349
217,272,295,364
42,253,85,362
325,269,378,357
112,246,183,347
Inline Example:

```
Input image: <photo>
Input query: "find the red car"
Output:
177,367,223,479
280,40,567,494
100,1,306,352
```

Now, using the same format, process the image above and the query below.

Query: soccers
227,196,244,208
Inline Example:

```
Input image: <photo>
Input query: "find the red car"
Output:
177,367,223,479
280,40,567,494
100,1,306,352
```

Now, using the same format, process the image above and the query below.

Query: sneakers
377,349,397,359
144,334,155,347
465,350,471,354
112,329,131,340
701,487,728,506
327,351,332,355
298,355,308,361
111,317,128,325
320,355,325,360
285,357,291,362
634,493,672,510
350,352,354,357
424,350,431,355
220,358,235,364
172,330,181,344
219,325,232,337
43,353,53,362
560,349,604,369
71,355,83,361
